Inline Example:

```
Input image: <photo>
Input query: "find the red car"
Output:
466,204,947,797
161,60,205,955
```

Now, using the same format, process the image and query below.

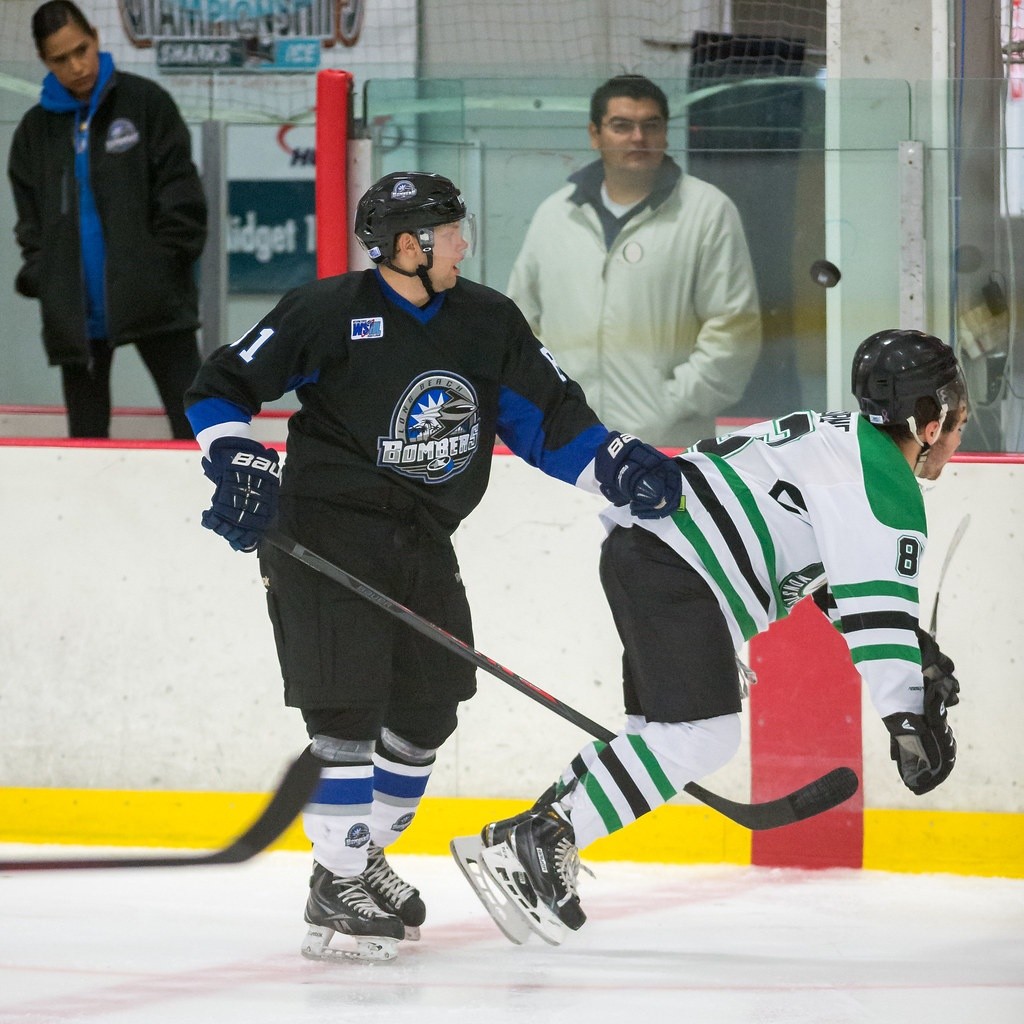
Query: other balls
808,256,842,289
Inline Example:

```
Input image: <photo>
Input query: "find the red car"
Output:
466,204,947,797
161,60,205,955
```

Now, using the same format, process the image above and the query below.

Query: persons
7,0,208,440
508,73,760,449
186,172,681,962
451,329,970,946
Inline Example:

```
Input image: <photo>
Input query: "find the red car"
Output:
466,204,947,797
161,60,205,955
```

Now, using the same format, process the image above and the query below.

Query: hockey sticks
1,741,323,873
262,529,862,834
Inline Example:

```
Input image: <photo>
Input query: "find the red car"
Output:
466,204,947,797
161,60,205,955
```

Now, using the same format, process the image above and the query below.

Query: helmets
850,328,968,426
352,170,468,265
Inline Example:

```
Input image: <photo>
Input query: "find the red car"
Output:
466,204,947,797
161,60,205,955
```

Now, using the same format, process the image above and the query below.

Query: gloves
915,629,960,708
880,676,958,796
200,436,281,552
594,430,683,519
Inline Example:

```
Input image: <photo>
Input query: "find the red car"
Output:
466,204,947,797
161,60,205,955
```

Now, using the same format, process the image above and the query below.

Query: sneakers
357,839,427,941
478,777,588,947
449,782,558,945
300,859,406,965
